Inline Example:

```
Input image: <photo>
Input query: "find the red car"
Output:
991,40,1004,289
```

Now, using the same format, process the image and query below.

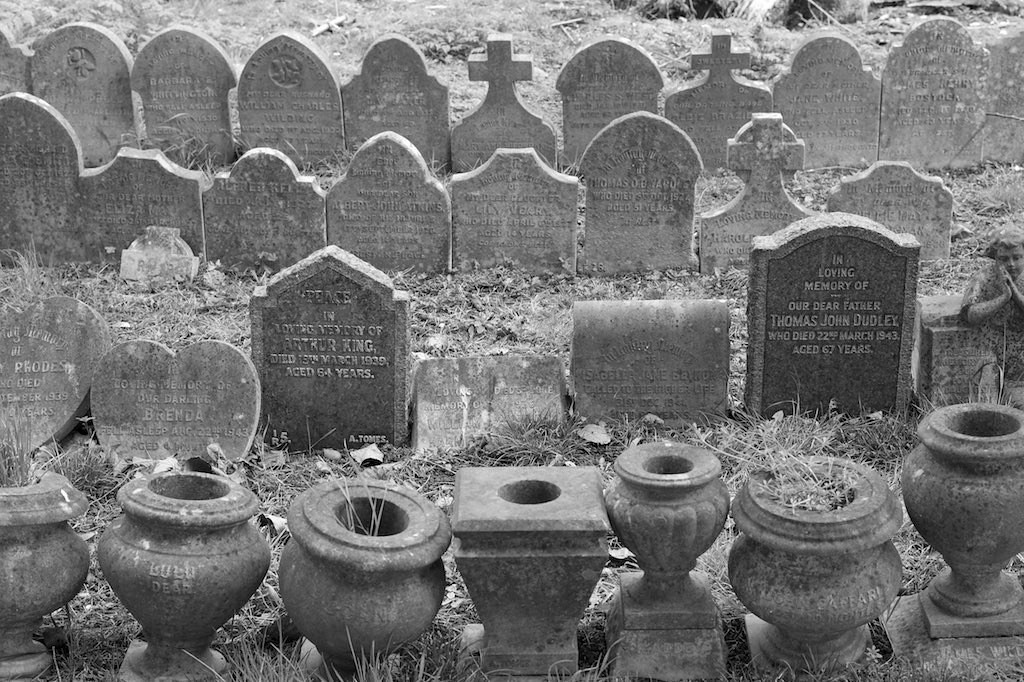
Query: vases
605,441,732,630
278,470,451,674
902,400,1024,616
91,470,273,682
449,464,616,674
730,451,903,668
0,467,94,676
